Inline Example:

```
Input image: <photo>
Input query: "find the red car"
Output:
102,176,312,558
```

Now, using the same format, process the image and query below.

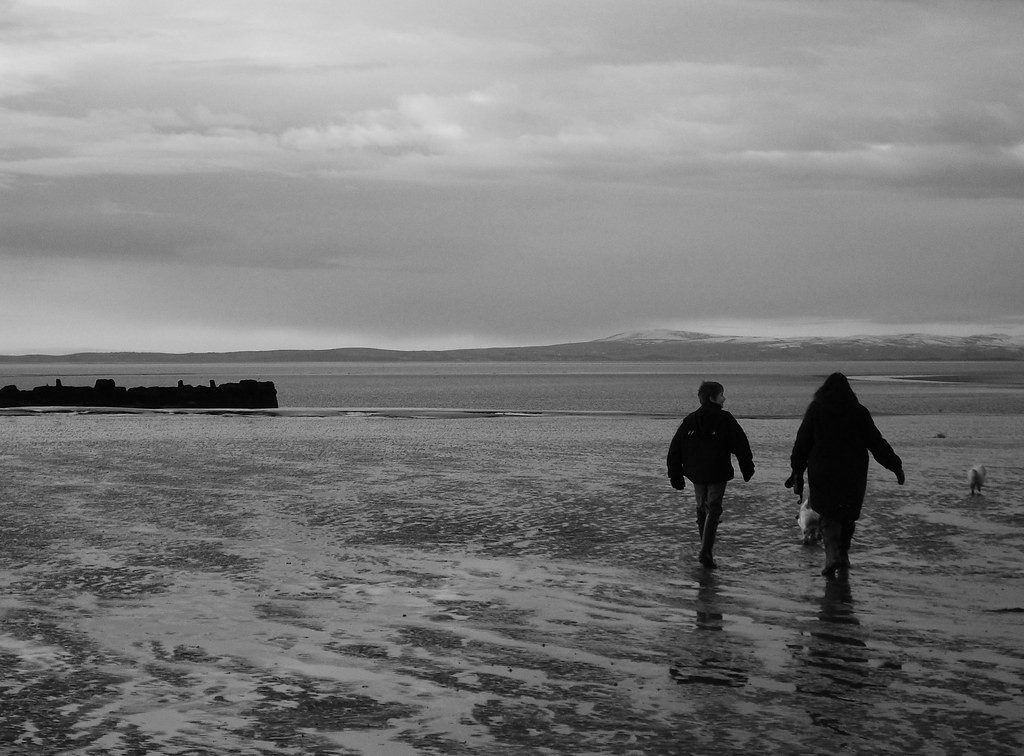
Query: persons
785,373,906,577
667,381,756,569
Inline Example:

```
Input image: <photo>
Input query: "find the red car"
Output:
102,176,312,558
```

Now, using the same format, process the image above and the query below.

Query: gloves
896,471,905,485
782,470,804,495
743,468,757,482
671,476,686,490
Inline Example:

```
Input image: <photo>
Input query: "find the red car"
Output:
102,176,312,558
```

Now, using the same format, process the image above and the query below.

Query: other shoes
699,554,716,568
822,558,852,575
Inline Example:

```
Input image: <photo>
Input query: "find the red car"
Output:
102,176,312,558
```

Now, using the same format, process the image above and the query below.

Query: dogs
795,499,822,544
968,463,986,495
